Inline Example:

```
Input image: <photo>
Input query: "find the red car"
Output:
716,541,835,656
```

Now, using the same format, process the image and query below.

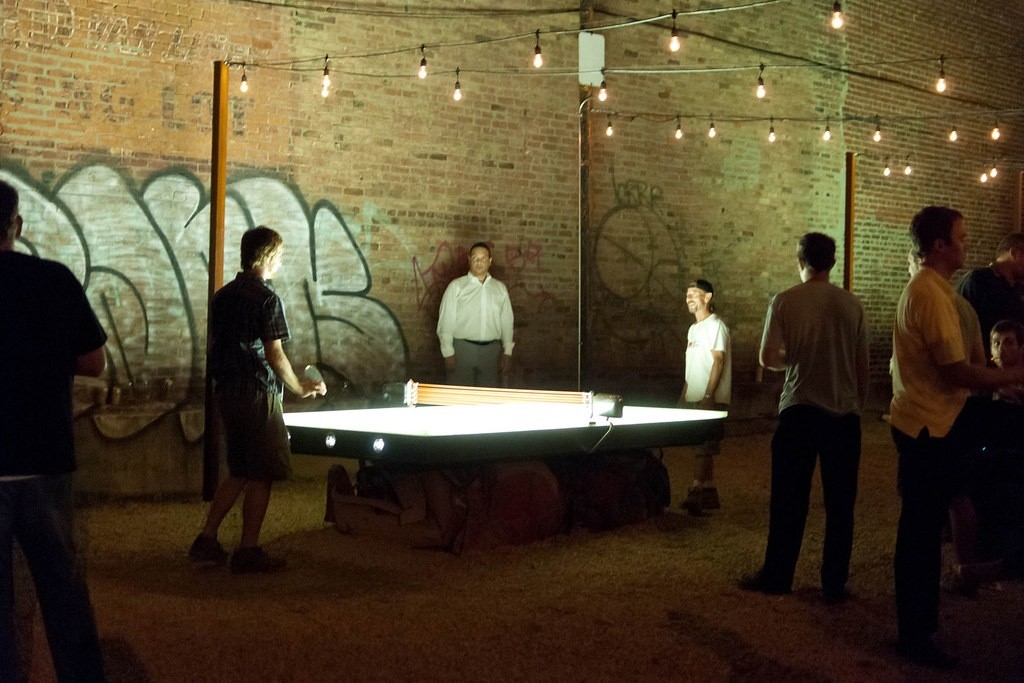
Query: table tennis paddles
304,365,327,397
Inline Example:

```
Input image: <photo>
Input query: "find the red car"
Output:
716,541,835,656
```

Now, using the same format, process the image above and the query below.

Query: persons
678,279,733,510
188,228,321,577
0,181,111,683
736,234,871,604
940,238,1024,594
436,243,515,387
883,203,1023,661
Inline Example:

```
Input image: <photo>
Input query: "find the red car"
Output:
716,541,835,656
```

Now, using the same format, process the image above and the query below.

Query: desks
285,379,730,557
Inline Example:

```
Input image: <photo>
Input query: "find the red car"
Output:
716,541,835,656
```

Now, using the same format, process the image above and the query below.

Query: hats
688,279,713,295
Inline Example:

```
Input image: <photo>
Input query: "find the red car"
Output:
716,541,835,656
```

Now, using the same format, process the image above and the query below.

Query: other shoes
743,572,790,595
823,586,844,602
680,487,720,510
189,533,228,561
232,545,288,571
688,488,702,515
897,639,961,668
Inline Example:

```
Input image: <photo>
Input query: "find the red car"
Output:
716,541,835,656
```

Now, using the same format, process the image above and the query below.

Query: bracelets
705,395,711,398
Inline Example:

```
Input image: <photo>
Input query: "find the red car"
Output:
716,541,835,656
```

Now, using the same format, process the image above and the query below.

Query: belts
465,339,494,345
